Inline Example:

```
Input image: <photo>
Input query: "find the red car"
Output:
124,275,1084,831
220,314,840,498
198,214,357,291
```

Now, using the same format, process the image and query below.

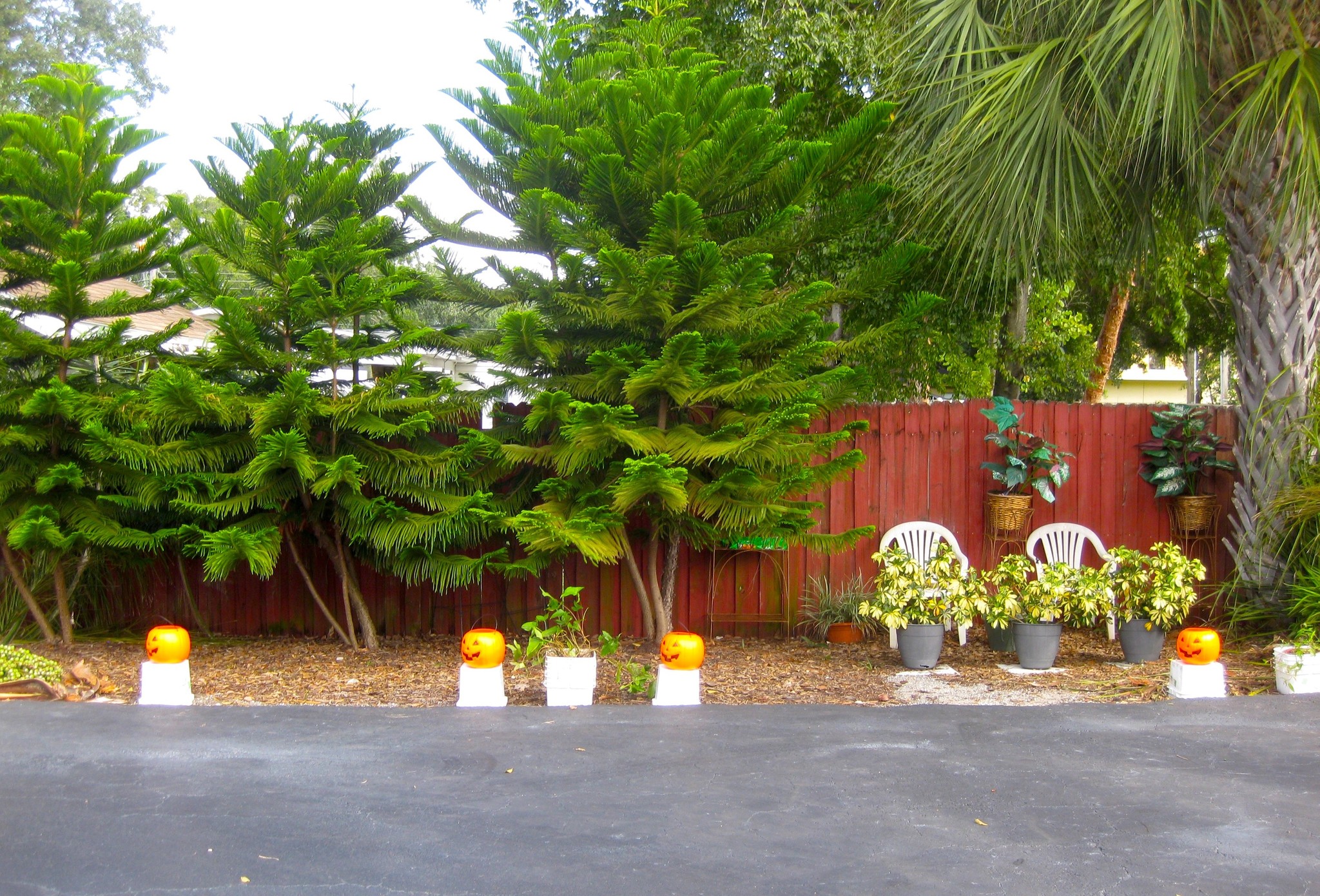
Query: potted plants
981,553,1018,655
793,569,888,646
978,547,1108,677
1135,399,1238,536
505,581,657,709
857,537,992,674
1105,539,1209,662
1263,620,1320,697
977,393,1077,532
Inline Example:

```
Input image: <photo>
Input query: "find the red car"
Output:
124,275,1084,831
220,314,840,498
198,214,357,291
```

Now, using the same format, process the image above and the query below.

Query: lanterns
1176,627,1219,665
146,625,191,663
659,632,703,670
462,628,506,668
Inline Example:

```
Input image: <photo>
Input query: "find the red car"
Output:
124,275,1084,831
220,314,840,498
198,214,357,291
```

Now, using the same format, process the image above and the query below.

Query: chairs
875,518,970,650
1025,522,1119,644
